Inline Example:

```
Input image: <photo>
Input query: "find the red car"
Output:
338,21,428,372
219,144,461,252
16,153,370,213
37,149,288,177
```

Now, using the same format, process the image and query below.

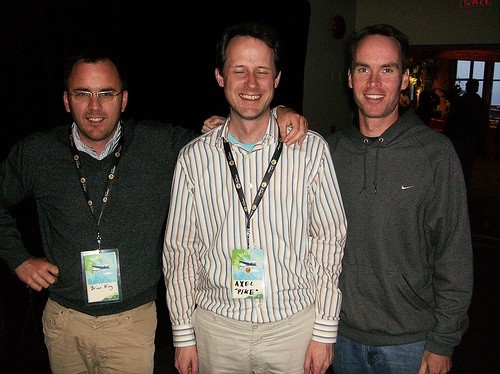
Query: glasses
64,88,124,103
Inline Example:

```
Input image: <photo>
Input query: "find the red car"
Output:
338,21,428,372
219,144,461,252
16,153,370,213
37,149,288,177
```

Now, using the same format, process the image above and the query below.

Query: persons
442,79,489,167
201,25,473,374
162,25,348,374
0,54,307,374
